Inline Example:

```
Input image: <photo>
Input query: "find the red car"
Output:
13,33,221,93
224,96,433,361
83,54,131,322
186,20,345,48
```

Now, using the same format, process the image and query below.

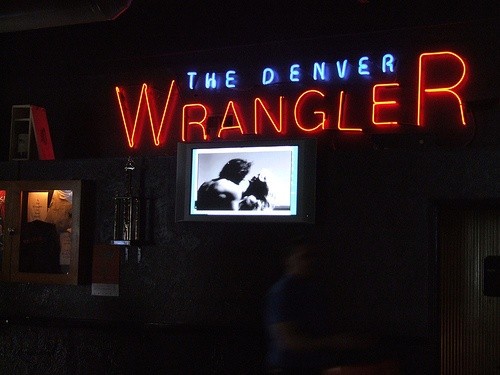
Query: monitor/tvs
175,137,319,226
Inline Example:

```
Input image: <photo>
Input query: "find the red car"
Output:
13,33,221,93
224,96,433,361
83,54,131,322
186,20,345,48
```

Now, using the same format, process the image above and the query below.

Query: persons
237,173,271,212
196,157,253,211
268,235,381,375
